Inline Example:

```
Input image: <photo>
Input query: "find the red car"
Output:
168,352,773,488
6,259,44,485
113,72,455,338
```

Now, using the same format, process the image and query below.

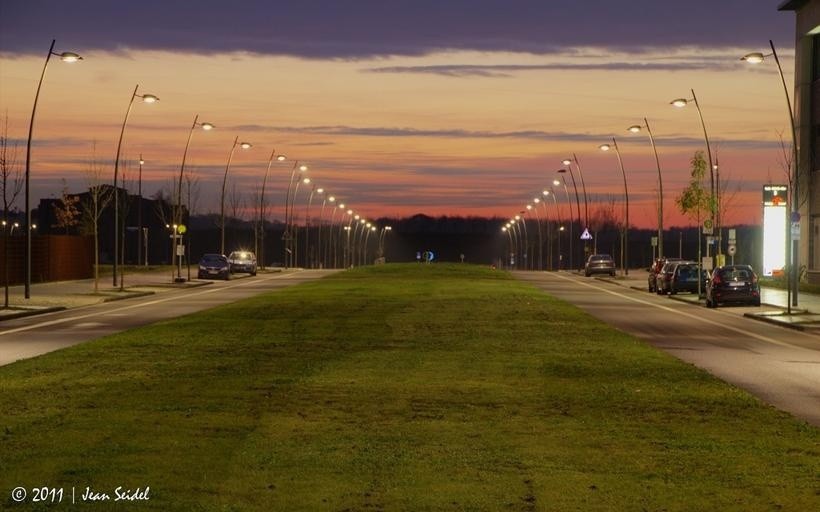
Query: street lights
253,147,394,272
101,82,161,289
500,151,594,274
738,40,803,308
597,133,630,279
625,118,669,267
171,114,216,284
667,87,725,274
217,135,252,259
19,38,82,302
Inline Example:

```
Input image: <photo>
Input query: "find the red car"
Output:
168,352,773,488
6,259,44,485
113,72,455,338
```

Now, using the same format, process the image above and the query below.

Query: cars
646,255,713,296
702,261,762,308
196,250,231,280
225,249,260,276
582,252,617,277
